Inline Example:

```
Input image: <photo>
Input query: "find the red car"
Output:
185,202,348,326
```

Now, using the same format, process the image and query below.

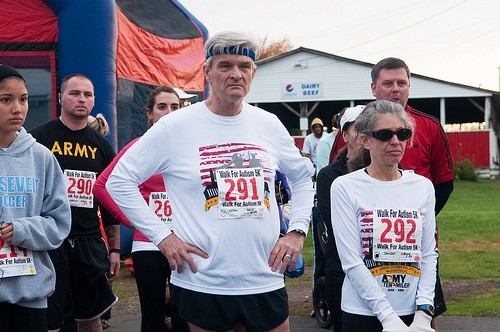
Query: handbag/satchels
280,220,304,279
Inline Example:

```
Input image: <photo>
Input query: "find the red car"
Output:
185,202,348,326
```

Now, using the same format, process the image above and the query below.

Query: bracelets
293,229,306,238
108,248,120,254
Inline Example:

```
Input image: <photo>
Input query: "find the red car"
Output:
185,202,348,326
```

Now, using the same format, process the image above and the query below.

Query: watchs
417,304,435,318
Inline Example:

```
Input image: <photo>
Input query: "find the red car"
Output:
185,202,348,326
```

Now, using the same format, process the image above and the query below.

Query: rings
285,254,291,258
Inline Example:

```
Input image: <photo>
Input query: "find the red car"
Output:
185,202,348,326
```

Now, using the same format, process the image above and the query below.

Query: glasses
367,128,412,142
181,101,192,107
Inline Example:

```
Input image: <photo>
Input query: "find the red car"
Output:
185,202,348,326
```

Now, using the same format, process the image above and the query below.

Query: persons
301,105,372,331
26,74,121,332
330,57,455,331
0,65,71,332
91,86,188,331
106,30,315,332
330,99,439,331
172,88,198,109
87,112,109,137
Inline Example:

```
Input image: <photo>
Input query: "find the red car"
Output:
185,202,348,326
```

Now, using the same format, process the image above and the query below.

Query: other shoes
101,320,108,329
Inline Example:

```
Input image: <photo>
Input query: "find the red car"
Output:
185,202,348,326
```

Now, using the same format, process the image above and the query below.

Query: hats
340,107,364,133
172,87,198,99
